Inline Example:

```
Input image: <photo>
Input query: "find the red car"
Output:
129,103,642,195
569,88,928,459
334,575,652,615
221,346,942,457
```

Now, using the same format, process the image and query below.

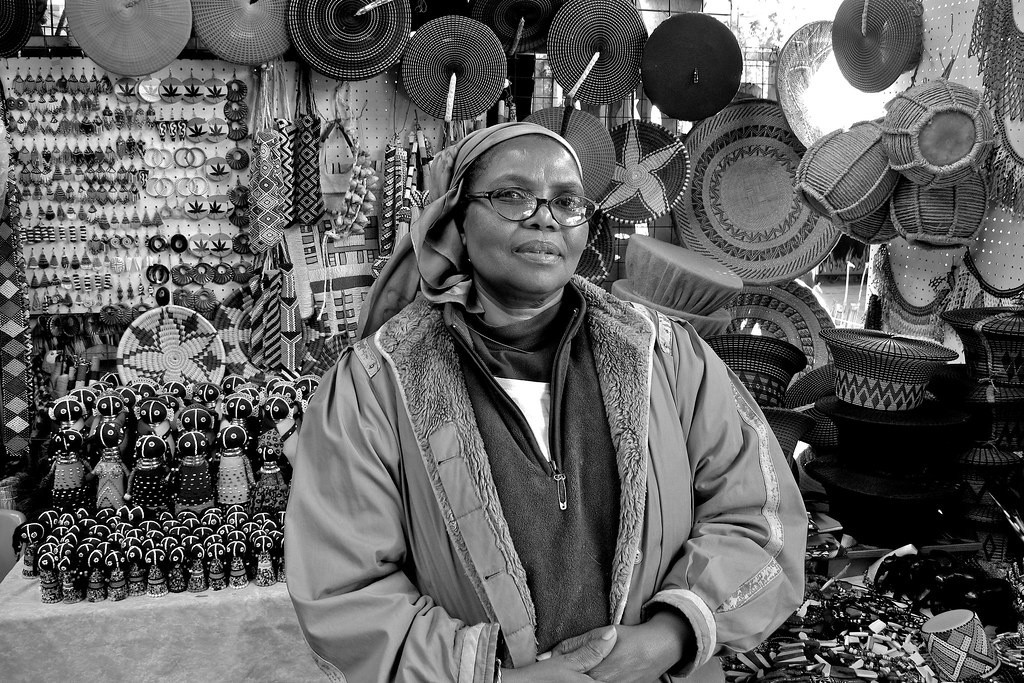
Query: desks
0,554,331,683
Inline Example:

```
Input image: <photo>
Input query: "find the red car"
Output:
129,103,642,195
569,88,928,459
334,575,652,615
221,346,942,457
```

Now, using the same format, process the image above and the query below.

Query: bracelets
144,146,208,197
494,658,502,683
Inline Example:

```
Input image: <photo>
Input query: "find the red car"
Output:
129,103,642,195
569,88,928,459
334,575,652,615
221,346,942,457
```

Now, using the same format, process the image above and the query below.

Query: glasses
462,185,599,227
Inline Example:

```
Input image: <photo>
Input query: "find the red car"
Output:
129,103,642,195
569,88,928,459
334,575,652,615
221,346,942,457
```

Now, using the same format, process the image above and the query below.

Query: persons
283,122,808,683
